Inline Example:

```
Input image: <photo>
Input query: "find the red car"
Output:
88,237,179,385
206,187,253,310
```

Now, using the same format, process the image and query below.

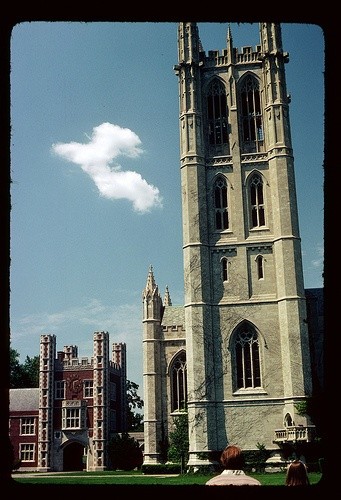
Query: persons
206,445,261,485
285,461,311,486
